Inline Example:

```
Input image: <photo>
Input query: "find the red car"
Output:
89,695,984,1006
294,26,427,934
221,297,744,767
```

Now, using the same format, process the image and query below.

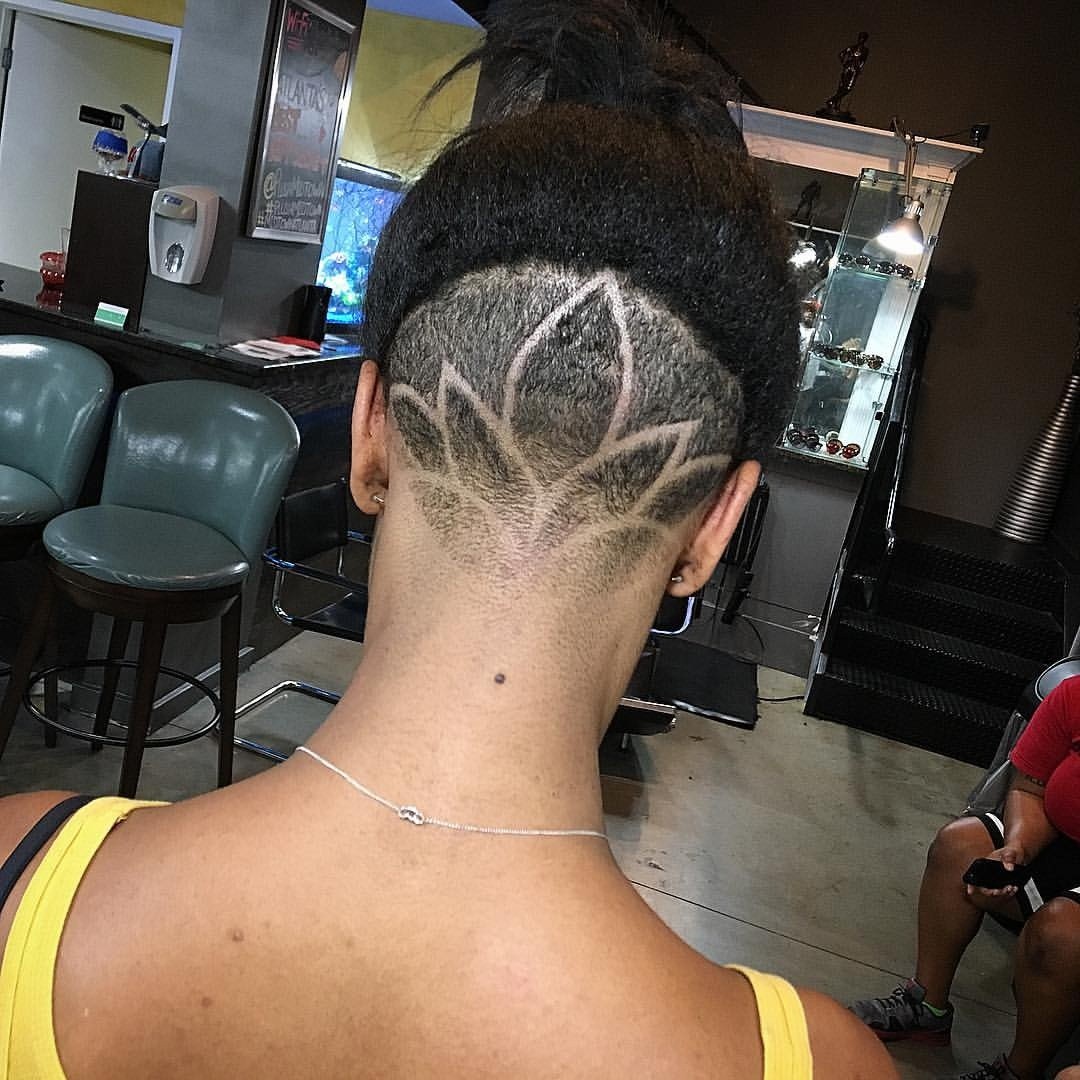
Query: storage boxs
314,156,417,335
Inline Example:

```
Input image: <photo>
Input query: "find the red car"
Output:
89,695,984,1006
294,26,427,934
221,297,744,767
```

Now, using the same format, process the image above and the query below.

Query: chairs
0,331,117,750
3,377,304,803
205,478,369,762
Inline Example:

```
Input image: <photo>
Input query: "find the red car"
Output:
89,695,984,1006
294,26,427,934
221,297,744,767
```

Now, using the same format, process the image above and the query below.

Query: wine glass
89,127,128,175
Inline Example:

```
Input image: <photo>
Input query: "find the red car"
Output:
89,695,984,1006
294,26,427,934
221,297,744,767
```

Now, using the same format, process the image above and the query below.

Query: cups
299,285,332,342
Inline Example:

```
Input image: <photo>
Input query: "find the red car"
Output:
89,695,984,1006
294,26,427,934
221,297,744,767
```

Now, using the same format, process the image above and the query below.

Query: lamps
865,113,932,256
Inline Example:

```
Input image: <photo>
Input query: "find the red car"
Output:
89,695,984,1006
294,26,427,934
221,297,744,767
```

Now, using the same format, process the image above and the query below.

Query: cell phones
962,858,1032,891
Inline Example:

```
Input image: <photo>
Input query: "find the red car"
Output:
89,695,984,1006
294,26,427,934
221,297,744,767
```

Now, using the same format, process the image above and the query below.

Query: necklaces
293,743,609,849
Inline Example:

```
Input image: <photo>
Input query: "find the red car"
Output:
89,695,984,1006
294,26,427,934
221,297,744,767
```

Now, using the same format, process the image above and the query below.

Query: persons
844,669,1080,1080
0,0,907,1080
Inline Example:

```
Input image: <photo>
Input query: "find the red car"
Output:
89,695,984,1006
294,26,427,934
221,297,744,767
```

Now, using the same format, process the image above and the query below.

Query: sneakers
959,1053,1018,1080
846,978,955,1046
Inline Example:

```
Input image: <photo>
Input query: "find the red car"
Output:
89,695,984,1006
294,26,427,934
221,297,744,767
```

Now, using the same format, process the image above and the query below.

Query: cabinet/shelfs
659,103,985,676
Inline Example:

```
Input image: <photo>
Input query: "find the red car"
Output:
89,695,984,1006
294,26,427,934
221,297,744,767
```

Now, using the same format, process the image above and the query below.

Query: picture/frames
246,0,358,247
